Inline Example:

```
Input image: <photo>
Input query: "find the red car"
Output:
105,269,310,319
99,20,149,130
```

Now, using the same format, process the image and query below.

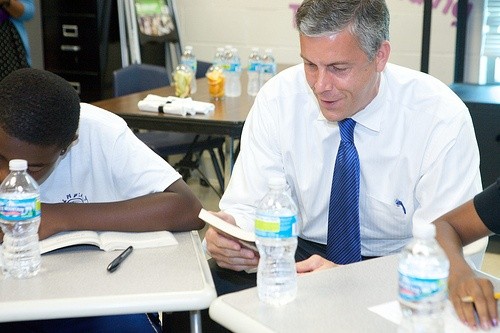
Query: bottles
215,44,241,98
180,45,197,94
397,221,449,333
0,159,42,280
254,175,299,306
246,46,277,96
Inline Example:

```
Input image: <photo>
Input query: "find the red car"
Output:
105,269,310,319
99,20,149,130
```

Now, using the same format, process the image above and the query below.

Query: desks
0,228,224,333
209,253,500,333
86,63,298,200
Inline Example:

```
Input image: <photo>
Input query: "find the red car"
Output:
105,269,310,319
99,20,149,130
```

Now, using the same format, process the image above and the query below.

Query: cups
206,65,225,101
175,65,191,98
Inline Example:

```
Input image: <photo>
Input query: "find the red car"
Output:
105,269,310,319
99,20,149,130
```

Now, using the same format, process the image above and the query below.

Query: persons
0,0,35,79
161,0,482,333
430,177,500,333
0,67,205,333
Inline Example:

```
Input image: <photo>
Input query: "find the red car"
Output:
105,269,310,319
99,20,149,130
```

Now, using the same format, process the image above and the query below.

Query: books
198,208,261,258
38,229,177,255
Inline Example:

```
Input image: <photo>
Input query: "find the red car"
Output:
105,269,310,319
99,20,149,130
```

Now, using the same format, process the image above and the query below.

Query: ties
326,118,361,266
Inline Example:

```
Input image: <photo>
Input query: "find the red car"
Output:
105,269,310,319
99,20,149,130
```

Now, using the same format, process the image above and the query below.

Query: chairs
114,60,243,186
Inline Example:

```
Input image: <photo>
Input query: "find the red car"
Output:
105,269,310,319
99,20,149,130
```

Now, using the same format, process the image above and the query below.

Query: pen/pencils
107,246,133,273
460,292,500,304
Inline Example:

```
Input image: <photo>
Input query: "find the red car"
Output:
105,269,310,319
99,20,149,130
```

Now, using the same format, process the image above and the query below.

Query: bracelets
0,0,11,8
